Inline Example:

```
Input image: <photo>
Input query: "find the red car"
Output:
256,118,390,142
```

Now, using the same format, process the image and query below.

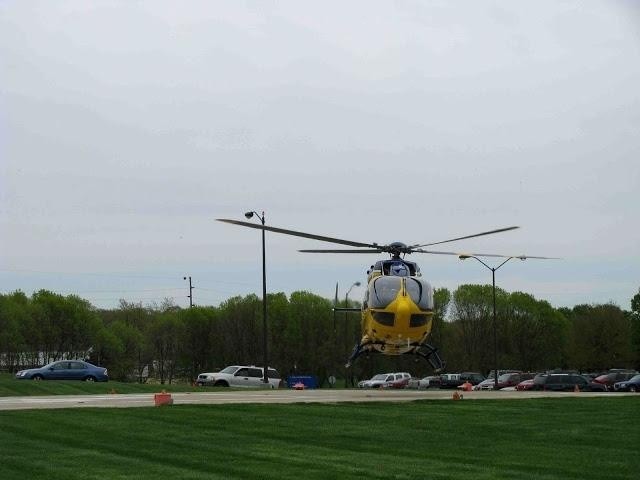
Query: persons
237,370,243,376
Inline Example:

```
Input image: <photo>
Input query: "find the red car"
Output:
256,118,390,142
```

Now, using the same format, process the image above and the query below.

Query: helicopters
215,219,520,373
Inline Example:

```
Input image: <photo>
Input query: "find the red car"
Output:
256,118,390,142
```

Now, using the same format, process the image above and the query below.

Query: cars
358,369,640,392
193,366,282,389
16,360,108,382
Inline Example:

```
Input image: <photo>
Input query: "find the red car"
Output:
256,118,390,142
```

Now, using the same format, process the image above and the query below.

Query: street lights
345,282,360,387
245,211,269,383
459,255,526,389
